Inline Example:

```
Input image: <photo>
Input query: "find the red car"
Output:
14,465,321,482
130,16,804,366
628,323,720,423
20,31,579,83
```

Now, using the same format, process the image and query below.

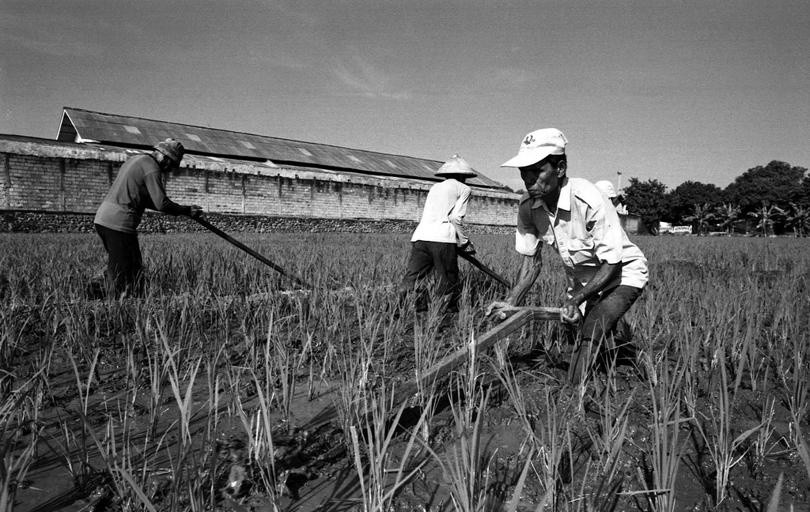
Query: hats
434,152,478,178
595,178,618,199
499,127,567,168
153,137,186,164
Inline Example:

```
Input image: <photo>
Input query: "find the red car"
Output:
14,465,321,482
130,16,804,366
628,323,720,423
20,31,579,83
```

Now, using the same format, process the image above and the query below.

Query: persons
93,136,203,300
550,178,619,253
483,127,650,386
384,152,478,317
